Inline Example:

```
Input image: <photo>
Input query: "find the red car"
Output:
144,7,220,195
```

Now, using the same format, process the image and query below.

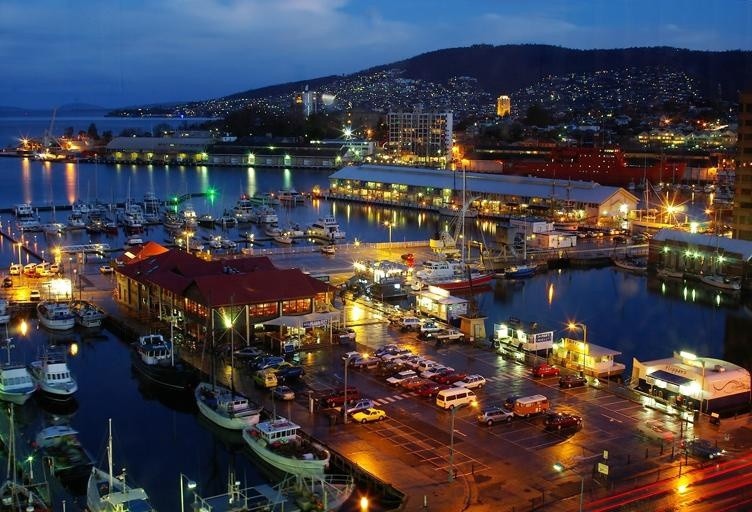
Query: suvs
322,385,361,407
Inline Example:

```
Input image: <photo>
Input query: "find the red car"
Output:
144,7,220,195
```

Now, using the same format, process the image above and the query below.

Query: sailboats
41,201,68,232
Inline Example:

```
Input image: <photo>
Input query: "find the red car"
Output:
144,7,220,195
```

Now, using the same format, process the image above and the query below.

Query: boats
629,179,713,232
614,259,648,271
15,216,42,232
13,203,34,217
70,169,345,250
0,296,11,325
351,250,543,301
549,338,626,379
195,380,264,430
0,324,39,407
190,463,354,511
30,342,78,402
38,283,75,330
84,420,155,511
701,273,742,291
73,276,104,328
493,317,555,356
124,233,144,246
656,266,686,278
132,319,186,390
37,422,96,477
438,206,478,219
1,403,53,512
503,142,688,188
243,416,332,479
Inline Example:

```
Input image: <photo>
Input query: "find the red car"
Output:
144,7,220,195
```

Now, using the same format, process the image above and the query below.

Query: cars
3,277,13,289
543,412,582,431
341,399,375,415
230,347,305,404
505,394,523,409
343,345,485,413
559,375,587,388
30,290,40,300
352,409,385,424
320,245,336,255
533,363,560,378
570,228,653,244
98,265,113,275
388,310,465,345
479,407,514,426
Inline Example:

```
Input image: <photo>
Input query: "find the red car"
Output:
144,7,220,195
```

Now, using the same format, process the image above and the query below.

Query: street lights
180,472,196,511
687,359,704,416
568,322,587,376
17,243,23,287
69,257,73,298
448,400,479,483
344,353,368,422
553,461,585,512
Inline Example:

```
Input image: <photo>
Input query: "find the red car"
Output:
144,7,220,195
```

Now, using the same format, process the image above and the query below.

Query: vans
9,263,64,278
513,393,549,417
